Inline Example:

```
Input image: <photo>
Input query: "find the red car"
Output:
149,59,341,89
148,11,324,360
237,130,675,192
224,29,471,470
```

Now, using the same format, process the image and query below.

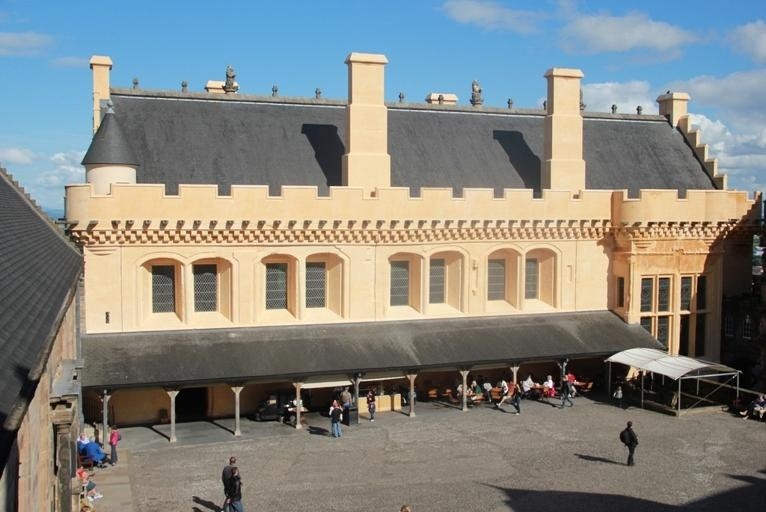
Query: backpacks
620,429,631,444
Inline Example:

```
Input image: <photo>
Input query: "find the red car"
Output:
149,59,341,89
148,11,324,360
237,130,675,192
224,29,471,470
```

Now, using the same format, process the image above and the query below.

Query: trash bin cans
345,405,358,426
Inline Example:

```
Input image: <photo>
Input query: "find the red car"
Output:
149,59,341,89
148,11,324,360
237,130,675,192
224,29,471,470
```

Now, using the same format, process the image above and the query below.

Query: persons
366,389,375,422
220,457,237,511
328,398,342,437
739,400,756,420
338,386,353,426
753,394,765,418
623,420,638,466
453,368,651,411
75,424,122,511
225,466,244,511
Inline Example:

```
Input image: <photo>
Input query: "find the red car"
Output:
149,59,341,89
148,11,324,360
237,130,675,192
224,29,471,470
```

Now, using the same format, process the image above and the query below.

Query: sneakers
94,494,103,499
88,496,94,502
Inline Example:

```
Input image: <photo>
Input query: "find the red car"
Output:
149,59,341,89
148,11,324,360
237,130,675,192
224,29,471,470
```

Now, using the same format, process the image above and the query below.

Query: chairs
426,382,569,408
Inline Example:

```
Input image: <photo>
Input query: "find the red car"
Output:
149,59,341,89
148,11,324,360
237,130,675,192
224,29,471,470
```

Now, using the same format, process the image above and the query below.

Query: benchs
79,450,93,471
